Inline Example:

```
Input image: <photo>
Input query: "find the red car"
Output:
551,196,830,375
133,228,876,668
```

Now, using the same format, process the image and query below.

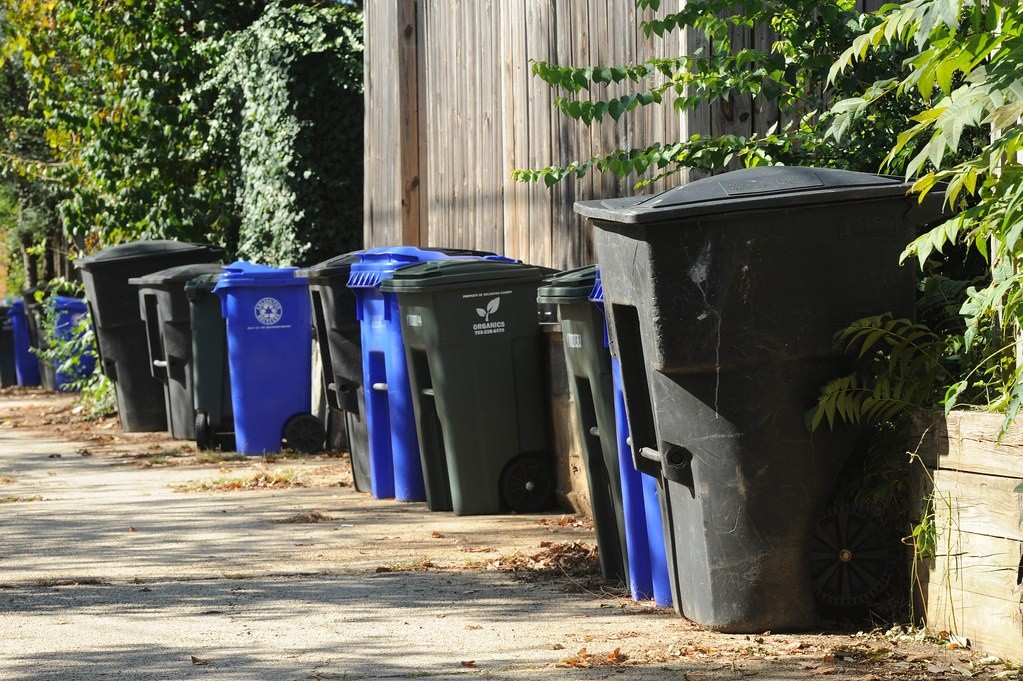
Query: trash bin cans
0,283,99,392
293,245,549,518
536,169,954,634
74,241,327,456
